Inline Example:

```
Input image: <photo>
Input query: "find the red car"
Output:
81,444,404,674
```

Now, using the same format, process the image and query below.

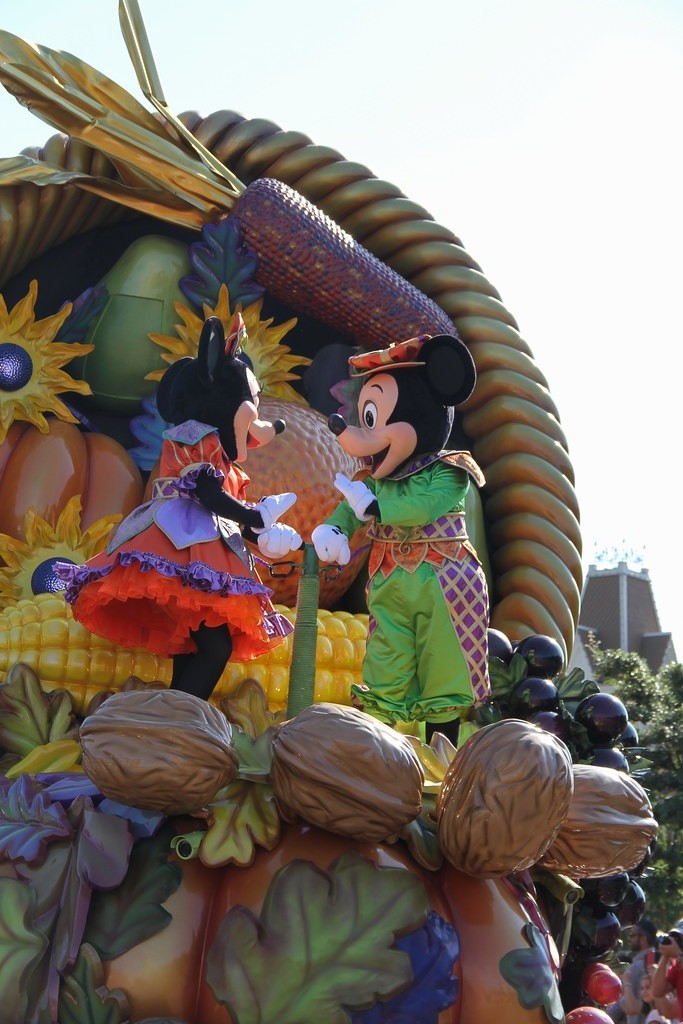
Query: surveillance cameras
529,869,584,905
171,829,207,860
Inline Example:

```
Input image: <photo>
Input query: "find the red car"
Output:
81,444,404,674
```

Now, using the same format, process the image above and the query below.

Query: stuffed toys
310,335,495,753
58,307,307,703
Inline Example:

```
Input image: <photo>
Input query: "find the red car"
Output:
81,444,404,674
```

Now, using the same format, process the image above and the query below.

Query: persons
601,916,683,1024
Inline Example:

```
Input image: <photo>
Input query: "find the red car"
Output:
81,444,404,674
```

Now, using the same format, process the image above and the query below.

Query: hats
668,919,683,935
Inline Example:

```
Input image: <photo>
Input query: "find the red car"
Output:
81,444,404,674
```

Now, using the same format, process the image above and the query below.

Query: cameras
656,931,679,946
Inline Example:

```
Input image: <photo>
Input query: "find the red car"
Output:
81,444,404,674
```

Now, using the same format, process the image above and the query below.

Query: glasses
628,932,646,938
